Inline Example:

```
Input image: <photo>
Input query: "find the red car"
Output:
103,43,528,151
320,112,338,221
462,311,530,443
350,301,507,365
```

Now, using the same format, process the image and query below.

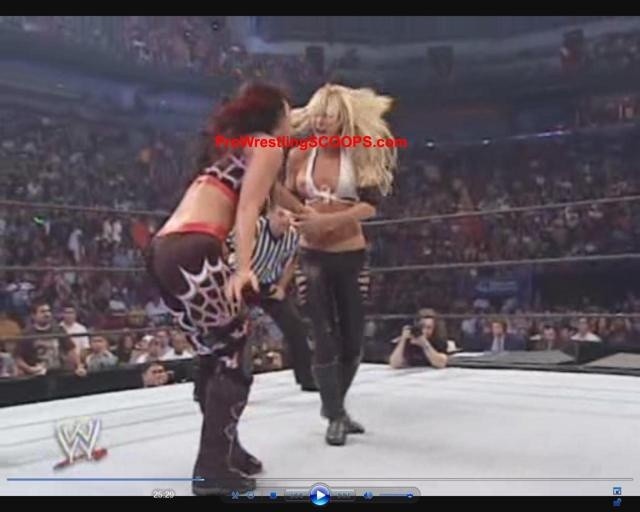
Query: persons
147,78,302,498
279,80,396,447
0,1,640,412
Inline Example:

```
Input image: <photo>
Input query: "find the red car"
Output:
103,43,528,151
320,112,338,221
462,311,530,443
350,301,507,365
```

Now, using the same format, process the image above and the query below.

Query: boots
310,354,364,444
192,360,262,495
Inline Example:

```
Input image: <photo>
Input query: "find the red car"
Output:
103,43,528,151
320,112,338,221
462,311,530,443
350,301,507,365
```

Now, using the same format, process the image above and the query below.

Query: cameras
410,324,423,337
